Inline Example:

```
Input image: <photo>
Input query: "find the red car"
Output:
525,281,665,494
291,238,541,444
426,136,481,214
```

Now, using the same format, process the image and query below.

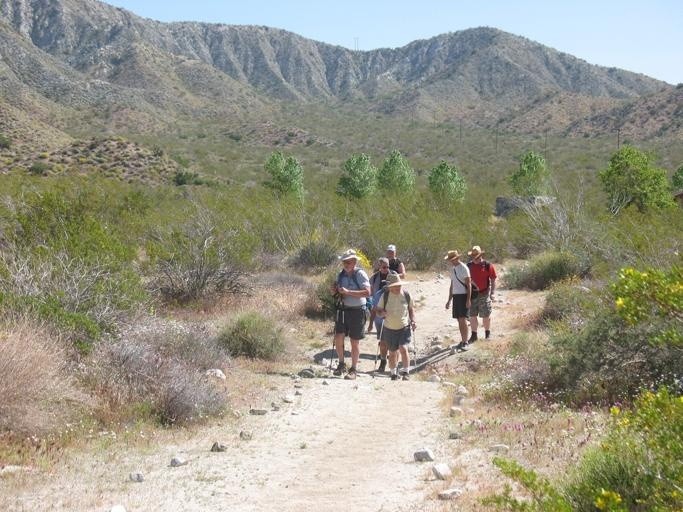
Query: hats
386,244,395,251
468,246,485,260
382,272,408,288
337,249,362,262
444,250,462,261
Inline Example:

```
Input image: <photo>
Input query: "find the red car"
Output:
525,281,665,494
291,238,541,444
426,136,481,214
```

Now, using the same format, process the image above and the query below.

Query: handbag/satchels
471,280,478,298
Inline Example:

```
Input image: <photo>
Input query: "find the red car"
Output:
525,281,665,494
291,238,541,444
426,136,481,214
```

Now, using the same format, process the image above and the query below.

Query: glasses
383,266,389,269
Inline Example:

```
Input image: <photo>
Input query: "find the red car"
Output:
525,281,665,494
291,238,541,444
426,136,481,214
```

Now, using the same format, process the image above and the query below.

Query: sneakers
333,361,346,376
454,336,477,351
390,367,409,380
379,360,387,371
345,367,357,380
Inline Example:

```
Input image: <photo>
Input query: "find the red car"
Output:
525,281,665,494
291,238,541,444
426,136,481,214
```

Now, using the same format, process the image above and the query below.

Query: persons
330,249,371,380
368,278,399,339
444,250,471,351
466,245,497,344
375,275,417,381
368,257,396,300
386,245,406,279
